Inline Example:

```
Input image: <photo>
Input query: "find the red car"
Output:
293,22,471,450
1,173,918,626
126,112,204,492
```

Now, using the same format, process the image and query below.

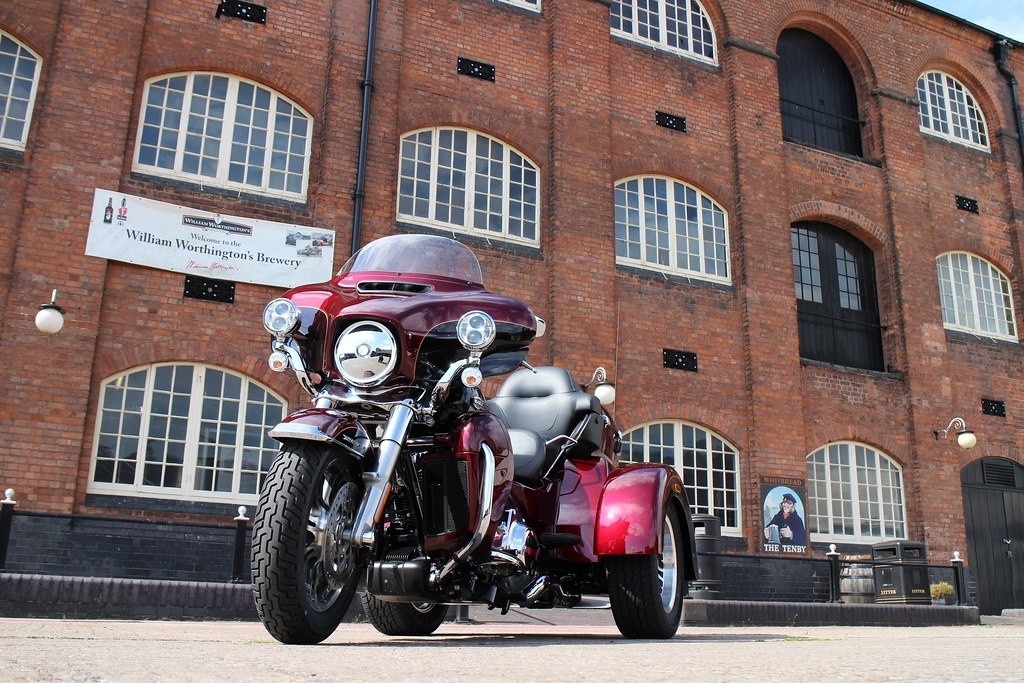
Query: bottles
104,198,114,224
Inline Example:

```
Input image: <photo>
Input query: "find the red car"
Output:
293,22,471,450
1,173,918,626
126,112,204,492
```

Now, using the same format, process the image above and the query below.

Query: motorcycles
250,233,700,647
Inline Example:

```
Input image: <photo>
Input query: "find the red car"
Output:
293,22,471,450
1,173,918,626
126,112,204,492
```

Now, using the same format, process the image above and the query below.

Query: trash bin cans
870,539,932,605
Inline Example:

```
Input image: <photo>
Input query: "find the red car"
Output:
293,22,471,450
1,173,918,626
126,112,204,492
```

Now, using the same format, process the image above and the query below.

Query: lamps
933,417,977,449
34,288,67,335
578,366,616,406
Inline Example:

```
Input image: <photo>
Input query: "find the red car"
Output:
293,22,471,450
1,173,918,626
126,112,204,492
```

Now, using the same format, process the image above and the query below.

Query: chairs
485,366,604,484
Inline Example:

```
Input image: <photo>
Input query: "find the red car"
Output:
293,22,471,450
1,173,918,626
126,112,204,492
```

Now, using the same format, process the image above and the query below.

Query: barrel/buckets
840,555,875,604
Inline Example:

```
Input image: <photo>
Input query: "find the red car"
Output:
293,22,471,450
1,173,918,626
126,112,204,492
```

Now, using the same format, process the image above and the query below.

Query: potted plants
930,580,957,605
